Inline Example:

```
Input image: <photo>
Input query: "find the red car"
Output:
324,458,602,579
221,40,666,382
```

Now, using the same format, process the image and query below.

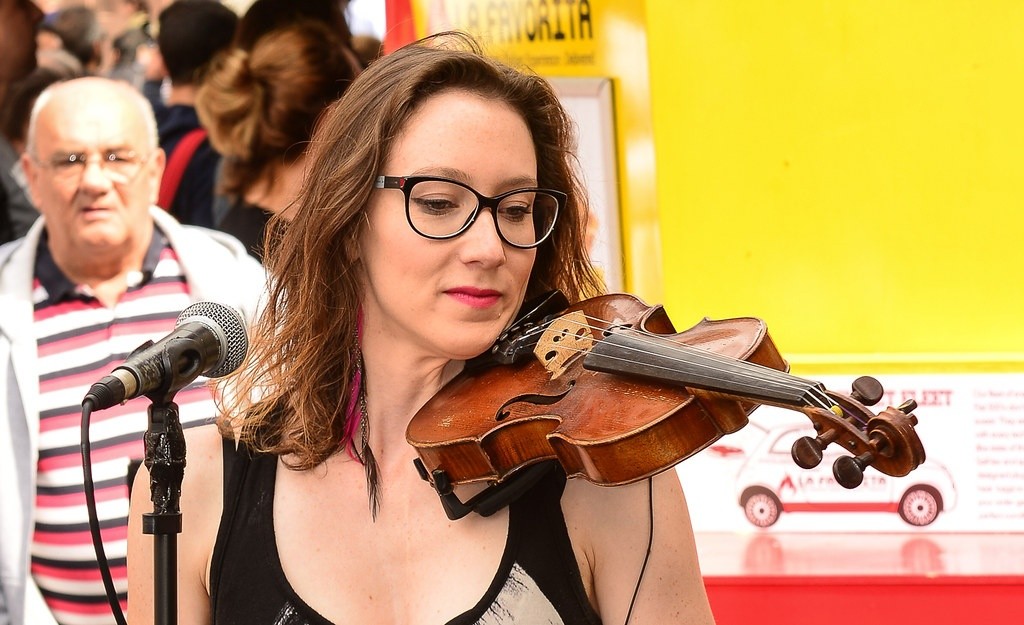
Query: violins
407,291,928,497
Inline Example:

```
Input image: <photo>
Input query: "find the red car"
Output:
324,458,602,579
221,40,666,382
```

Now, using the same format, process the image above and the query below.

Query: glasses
375,176,569,250
27,144,154,184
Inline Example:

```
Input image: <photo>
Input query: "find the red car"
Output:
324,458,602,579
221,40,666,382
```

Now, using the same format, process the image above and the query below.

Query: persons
127,32,722,625
0,0,380,625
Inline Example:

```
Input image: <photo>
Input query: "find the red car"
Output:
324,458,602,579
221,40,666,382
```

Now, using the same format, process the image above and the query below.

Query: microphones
81,301,249,412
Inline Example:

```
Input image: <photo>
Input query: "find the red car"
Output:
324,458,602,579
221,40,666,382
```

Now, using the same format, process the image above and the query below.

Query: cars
733,423,959,528
